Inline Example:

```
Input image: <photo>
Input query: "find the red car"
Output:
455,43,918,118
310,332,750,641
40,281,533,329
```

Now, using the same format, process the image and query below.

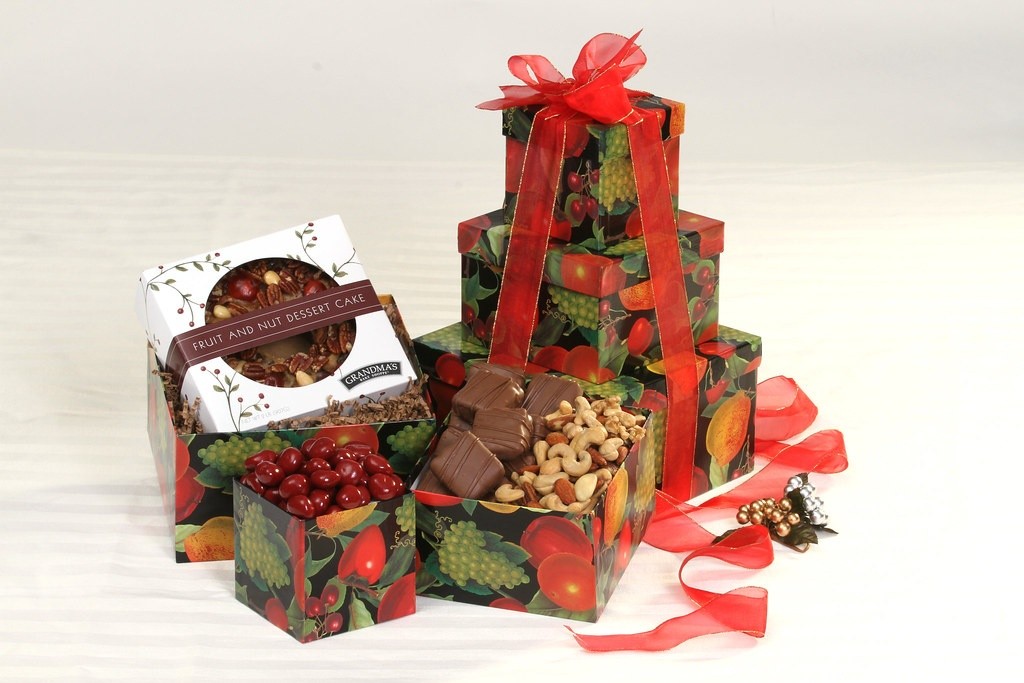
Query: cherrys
240,438,406,521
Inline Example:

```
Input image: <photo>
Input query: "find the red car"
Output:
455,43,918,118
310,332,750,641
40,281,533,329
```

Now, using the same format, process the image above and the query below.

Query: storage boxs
411,322,762,512
458,209,725,386
502,86,686,251
411,404,656,627
137,293,434,563
140,213,417,433
232,476,417,645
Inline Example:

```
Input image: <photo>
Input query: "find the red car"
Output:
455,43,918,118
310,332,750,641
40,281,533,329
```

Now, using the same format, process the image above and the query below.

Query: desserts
415,363,583,502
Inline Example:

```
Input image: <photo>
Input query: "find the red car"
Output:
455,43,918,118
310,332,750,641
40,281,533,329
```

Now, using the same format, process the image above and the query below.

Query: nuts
204,260,354,387
495,395,646,512
